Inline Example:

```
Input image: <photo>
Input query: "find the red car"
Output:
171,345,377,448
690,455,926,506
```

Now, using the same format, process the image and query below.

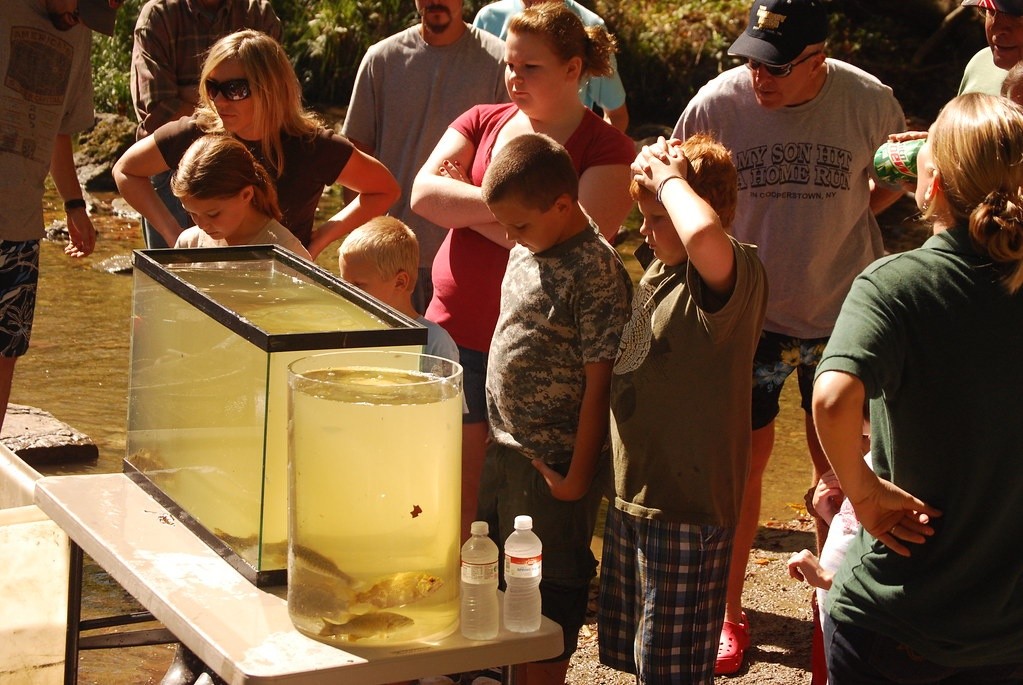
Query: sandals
713,611,749,673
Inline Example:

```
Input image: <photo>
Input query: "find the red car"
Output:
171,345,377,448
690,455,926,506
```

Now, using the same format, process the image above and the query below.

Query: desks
35,473,564,684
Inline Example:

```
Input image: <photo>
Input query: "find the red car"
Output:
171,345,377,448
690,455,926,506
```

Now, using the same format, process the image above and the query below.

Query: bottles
504,515,542,631
461,521,499,641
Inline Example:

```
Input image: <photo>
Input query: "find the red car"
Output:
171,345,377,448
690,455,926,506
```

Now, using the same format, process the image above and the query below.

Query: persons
666,0,907,674
0,0,639,685
787,0,1023,685
595,126,769,685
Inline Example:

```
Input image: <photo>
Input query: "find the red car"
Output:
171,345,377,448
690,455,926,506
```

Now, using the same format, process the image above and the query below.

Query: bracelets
64,199,87,211
654,170,683,203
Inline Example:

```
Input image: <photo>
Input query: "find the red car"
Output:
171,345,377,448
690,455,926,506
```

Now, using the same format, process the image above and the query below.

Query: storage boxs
121,245,428,587
0,441,71,673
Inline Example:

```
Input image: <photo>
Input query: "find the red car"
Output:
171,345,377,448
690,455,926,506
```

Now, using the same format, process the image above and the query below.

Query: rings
890,525,897,534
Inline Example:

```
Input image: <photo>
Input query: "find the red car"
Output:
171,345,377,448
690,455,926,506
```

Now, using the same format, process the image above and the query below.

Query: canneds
873,138,926,184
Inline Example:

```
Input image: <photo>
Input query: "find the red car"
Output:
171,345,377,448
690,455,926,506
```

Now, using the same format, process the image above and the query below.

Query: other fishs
292,543,363,590
316,610,415,642
263,539,288,560
350,571,445,609
214,529,259,555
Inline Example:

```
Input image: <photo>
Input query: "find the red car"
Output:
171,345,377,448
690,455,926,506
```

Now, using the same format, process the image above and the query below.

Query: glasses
749,50,826,77
205,71,260,102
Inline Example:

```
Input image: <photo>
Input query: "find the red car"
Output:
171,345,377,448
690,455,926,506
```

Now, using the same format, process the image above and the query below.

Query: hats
77,0,123,36
960,0,1023,12
727,0,828,66
